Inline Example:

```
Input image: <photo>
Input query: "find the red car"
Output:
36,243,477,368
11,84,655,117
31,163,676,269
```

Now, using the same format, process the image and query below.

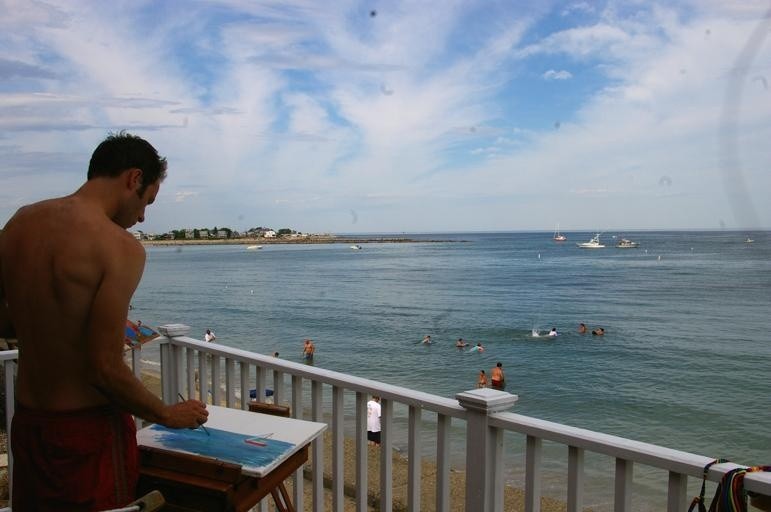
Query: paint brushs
177,392,210,437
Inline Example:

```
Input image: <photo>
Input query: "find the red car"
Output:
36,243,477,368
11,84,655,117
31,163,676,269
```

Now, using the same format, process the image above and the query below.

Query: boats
554,223,567,242
615,239,640,248
576,232,606,249
247,245,262,250
350,245,361,250
743,237,754,243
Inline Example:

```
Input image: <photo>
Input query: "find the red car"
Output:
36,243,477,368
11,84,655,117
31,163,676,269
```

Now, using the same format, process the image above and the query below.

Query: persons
477,369,488,387
581,323,588,333
303,337,314,360
368,394,382,446
0,133,209,510
421,335,433,343
550,327,560,336
272,350,280,358
491,361,506,388
591,326,607,335
456,337,470,346
206,329,216,342
471,342,484,352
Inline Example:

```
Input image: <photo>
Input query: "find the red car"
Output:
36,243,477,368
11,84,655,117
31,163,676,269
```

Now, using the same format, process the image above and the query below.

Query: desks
140,401,311,512
